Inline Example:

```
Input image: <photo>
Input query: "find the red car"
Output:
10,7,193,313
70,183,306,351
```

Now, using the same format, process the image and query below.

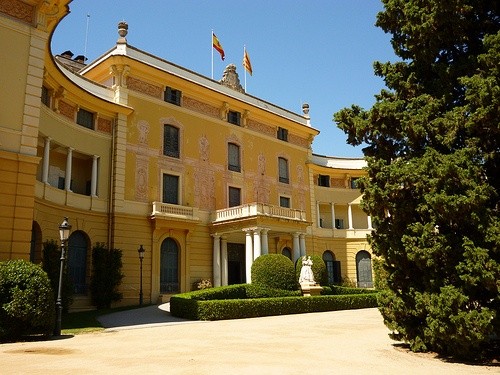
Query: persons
307,255,316,284
298,255,310,285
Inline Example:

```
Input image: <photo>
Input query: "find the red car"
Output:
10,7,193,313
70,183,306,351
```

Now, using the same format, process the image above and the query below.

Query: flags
244,49,253,76
212,33,225,61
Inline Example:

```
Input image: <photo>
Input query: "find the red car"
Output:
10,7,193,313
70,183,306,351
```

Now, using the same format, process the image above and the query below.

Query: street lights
138,244,146,306
53,216,73,336
